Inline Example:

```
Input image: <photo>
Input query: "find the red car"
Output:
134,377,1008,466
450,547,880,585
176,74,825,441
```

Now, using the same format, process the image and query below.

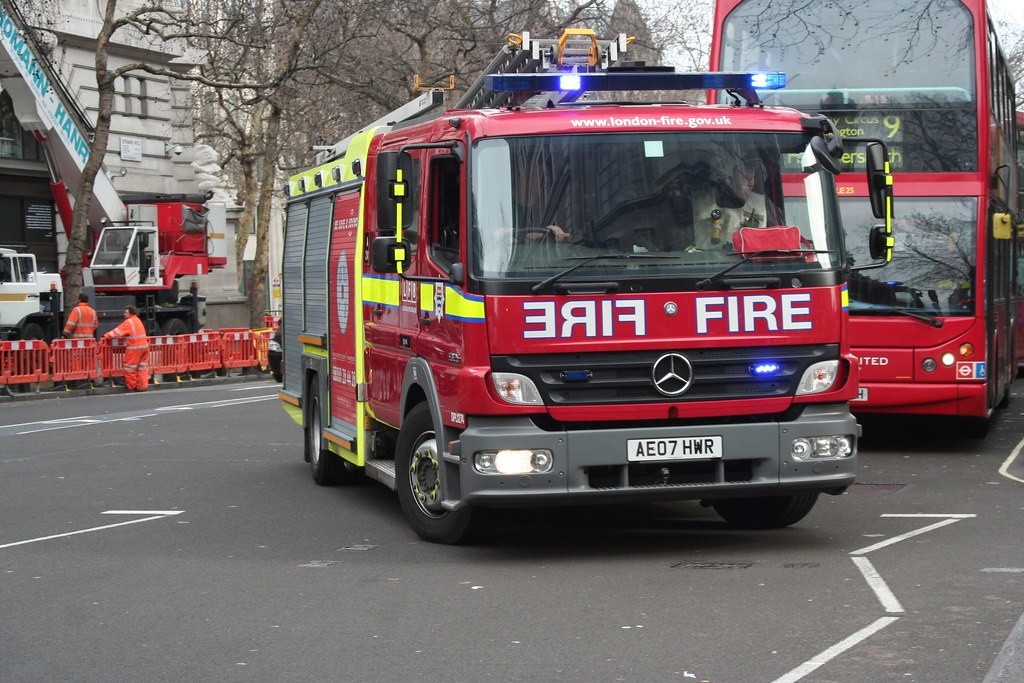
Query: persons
512,174,570,243
99,306,150,391
675,164,779,251
60,293,98,390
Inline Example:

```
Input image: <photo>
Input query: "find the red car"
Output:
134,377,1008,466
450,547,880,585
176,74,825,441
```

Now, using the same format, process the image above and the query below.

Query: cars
268,325,284,382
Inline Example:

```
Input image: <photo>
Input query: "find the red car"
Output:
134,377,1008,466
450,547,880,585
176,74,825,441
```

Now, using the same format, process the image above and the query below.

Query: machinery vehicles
0,0,228,374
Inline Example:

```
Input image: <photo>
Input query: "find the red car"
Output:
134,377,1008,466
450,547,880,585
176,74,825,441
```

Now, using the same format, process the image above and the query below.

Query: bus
710,1,1024,423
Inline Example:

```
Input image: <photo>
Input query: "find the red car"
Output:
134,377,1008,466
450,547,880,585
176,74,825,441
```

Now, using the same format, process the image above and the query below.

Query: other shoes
127,389,135,392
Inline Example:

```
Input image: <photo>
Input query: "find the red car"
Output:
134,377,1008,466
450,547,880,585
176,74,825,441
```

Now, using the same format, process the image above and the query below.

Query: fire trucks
276,24,896,546
